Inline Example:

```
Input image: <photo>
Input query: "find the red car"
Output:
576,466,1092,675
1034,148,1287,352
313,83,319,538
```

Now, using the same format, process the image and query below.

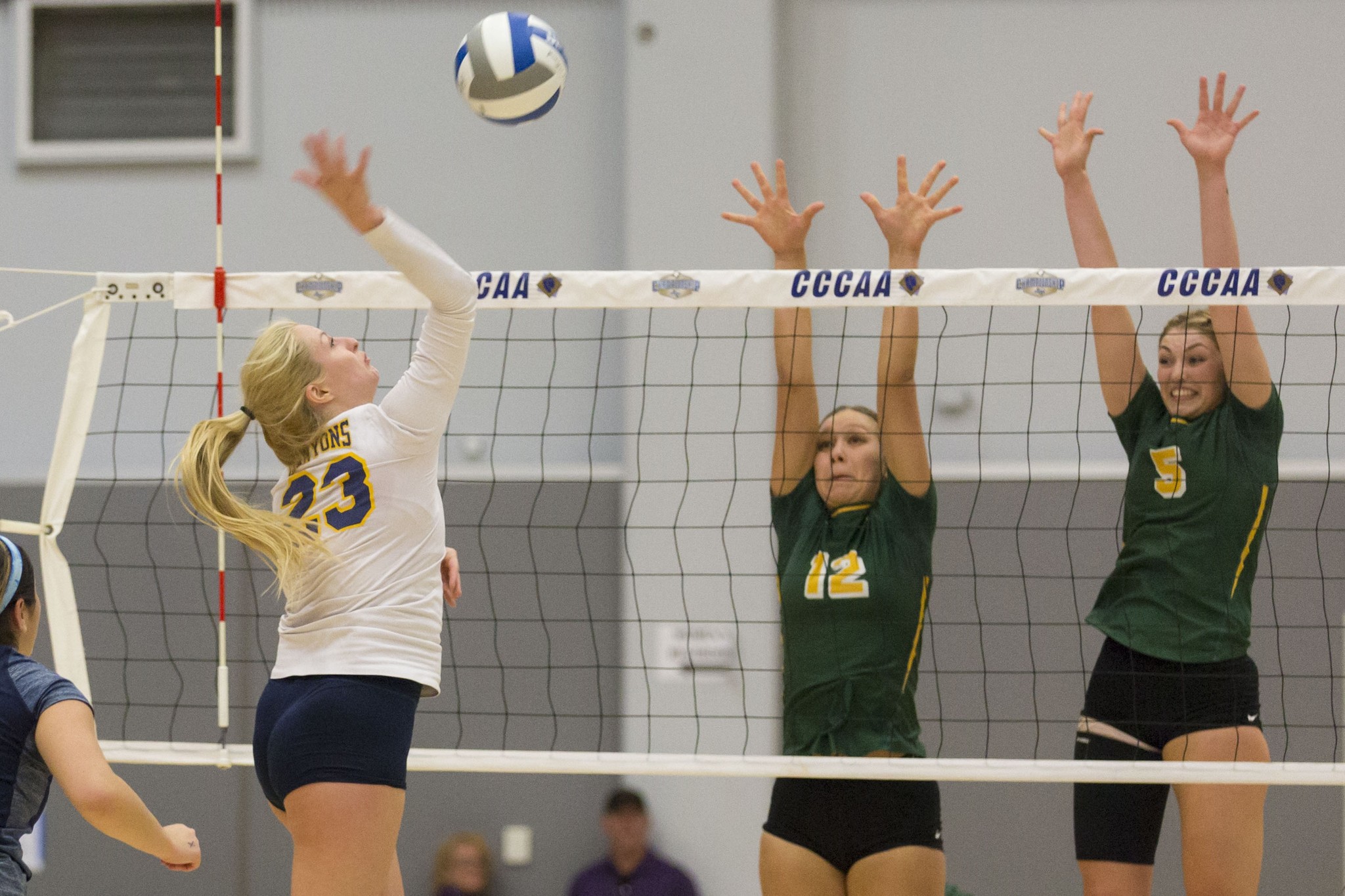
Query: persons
0,533,202,896
1033,70,1286,896
716,155,966,895
175,128,478,896
566,791,697,896
434,831,503,896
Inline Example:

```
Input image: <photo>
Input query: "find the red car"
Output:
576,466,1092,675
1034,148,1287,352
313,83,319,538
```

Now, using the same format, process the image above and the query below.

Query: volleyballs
451,10,570,127
